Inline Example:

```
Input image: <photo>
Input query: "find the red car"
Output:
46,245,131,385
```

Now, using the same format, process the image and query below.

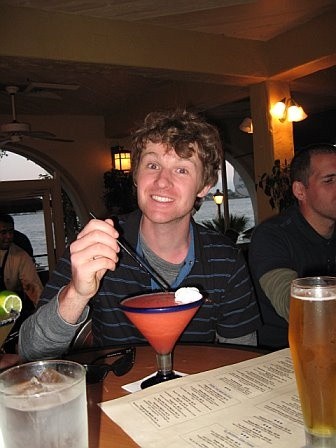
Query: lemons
0,291,23,315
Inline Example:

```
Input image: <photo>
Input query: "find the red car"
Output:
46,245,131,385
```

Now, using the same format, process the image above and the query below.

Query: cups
0,312,18,351
288,274,336,448
0,358,89,448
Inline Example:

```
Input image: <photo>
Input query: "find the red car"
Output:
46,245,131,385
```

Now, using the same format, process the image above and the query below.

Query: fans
0,86,75,143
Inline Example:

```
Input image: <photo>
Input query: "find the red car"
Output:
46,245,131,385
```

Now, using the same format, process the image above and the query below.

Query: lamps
270,97,308,123
240,117,253,133
112,147,131,171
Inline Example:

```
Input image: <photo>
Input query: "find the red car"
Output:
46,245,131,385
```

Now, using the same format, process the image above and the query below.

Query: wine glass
119,287,206,390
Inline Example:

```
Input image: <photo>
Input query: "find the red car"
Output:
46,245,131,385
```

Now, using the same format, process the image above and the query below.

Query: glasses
84,346,136,385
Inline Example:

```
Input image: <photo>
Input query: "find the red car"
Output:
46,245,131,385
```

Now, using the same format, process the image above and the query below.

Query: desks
0,341,336,448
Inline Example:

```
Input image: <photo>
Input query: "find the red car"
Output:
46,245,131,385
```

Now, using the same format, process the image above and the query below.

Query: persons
247,142,336,352
0,213,45,309
19,110,262,364
